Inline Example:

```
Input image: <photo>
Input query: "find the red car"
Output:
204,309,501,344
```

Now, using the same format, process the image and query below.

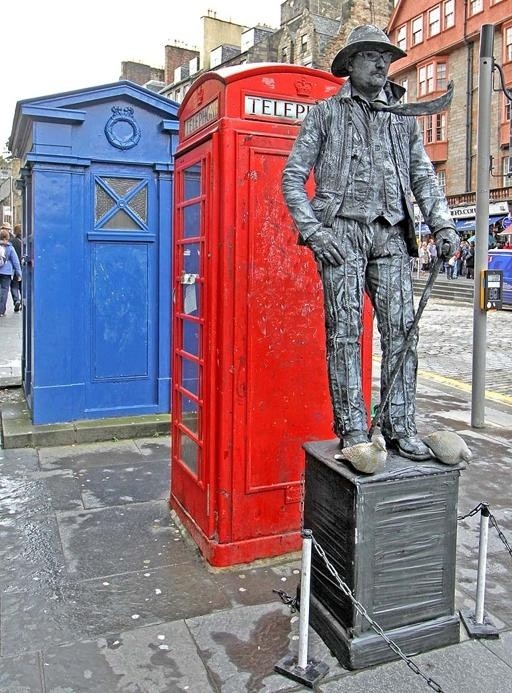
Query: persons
279,23,459,475
11,223,24,311
1,230,23,316
409,230,511,279
1,220,22,279
